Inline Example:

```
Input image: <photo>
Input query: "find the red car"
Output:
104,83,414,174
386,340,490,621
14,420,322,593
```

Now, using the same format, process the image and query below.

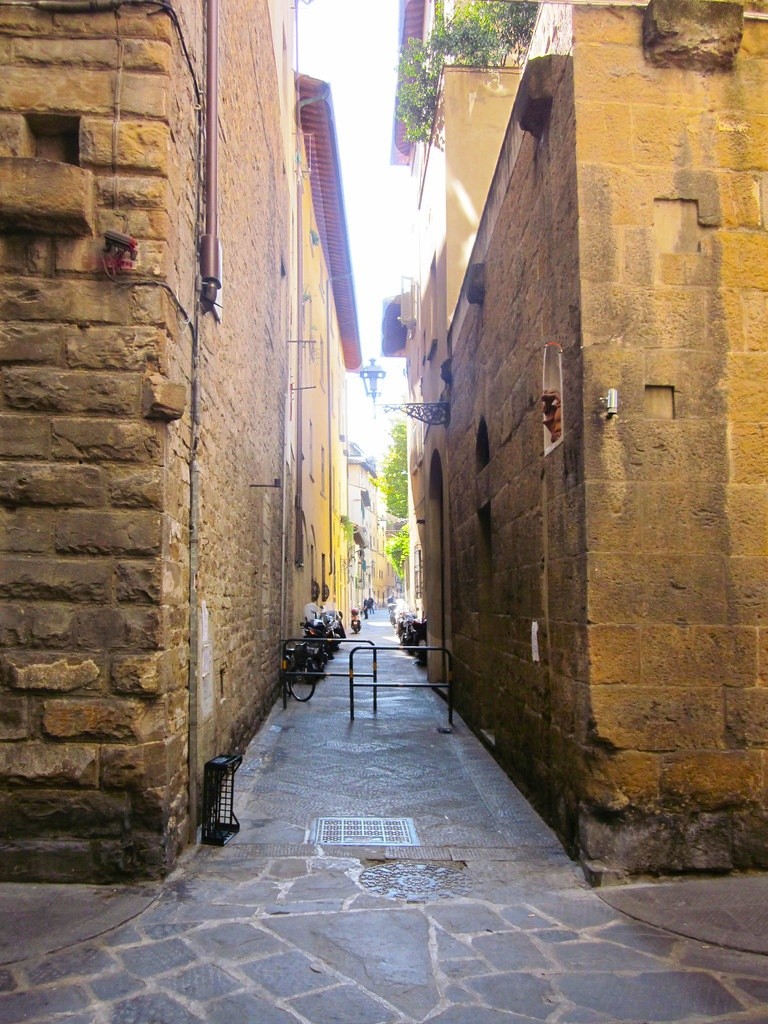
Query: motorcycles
350,607,363,632
298,599,345,680
388,604,426,650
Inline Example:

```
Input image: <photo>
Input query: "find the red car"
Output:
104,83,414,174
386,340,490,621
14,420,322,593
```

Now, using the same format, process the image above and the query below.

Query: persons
362,595,377,619
388,594,393,613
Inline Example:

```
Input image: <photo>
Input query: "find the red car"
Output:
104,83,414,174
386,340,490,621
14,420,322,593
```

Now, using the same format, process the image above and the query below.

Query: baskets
201,755,242,846
